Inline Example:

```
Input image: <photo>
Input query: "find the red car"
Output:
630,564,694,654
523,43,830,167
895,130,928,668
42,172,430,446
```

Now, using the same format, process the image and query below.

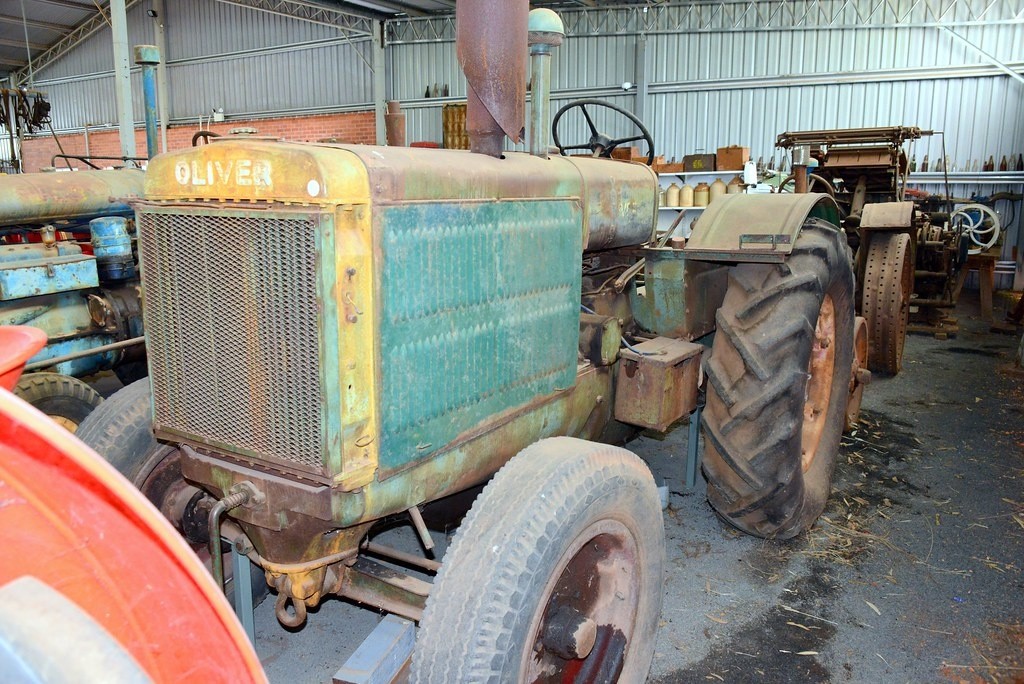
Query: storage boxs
613,337,703,433
717,147,748,170
657,164,684,172
632,157,666,172
611,147,639,160
684,154,716,171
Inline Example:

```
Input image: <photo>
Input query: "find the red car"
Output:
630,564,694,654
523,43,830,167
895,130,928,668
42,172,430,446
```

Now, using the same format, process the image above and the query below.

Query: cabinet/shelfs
657,162,757,239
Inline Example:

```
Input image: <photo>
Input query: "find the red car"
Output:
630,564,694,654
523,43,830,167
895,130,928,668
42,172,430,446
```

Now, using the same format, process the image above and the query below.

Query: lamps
212,108,224,122
622,82,631,92
147,9,164,28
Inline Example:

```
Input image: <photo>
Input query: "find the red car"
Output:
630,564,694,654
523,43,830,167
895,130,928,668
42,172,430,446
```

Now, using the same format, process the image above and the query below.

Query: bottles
983,161,988,171
694,182,709,206
728,174,743,193
936,159,942,172
972,160,979,172
679,184,694,206
965,160,970,172
1000,155,1007,171
1016,153,1024,171
710,177,726,203
769,157,774,170
988,156,994,171
659,184,666,207
921,156,928,172
910,159,916,172
780,156,786,172
757,157,763,169
1008,156,1015,170
667,183,680,207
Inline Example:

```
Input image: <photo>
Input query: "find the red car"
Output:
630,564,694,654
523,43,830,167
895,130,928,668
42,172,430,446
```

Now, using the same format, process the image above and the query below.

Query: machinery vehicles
0,0,856,684
771,121,963,374
0,42,225,441
